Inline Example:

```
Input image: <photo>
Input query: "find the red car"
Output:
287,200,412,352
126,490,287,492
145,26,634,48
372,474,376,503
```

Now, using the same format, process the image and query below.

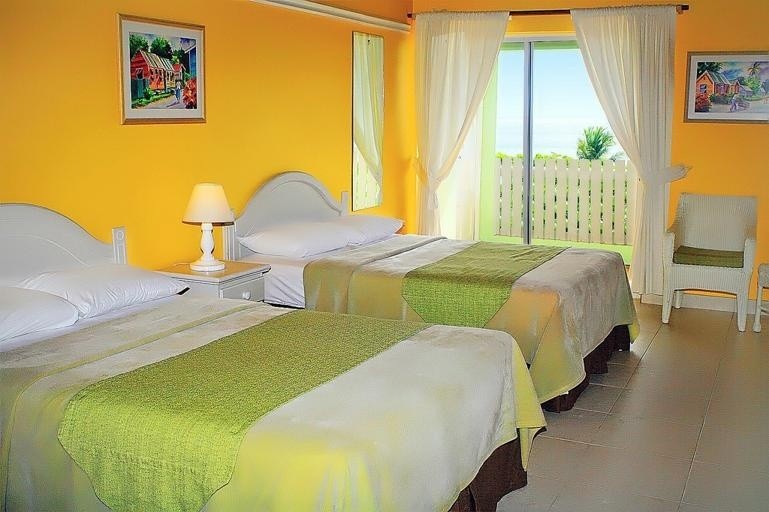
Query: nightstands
154,259,272,307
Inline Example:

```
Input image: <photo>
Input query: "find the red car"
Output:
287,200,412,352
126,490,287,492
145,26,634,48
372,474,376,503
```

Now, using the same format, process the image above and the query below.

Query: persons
175,79,181,101
728,92,739,112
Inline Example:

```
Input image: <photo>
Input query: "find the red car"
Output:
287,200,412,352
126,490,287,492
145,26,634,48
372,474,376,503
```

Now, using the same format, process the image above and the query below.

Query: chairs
658,192,758,333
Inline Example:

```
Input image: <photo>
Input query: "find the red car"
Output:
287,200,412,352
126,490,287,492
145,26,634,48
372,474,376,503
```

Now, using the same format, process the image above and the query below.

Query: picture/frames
683,50,769,125
117,12,206,126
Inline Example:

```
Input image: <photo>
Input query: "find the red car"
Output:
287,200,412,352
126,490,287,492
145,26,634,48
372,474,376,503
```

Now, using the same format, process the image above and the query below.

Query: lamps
182,181,235,273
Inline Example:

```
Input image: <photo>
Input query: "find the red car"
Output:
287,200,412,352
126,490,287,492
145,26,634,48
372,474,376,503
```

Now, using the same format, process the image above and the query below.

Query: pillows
0,263,189,341
238,215,404,256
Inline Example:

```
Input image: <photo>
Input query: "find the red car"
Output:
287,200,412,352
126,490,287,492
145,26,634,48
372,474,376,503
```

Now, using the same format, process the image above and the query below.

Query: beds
223,173,622,413
1,201,524,510
